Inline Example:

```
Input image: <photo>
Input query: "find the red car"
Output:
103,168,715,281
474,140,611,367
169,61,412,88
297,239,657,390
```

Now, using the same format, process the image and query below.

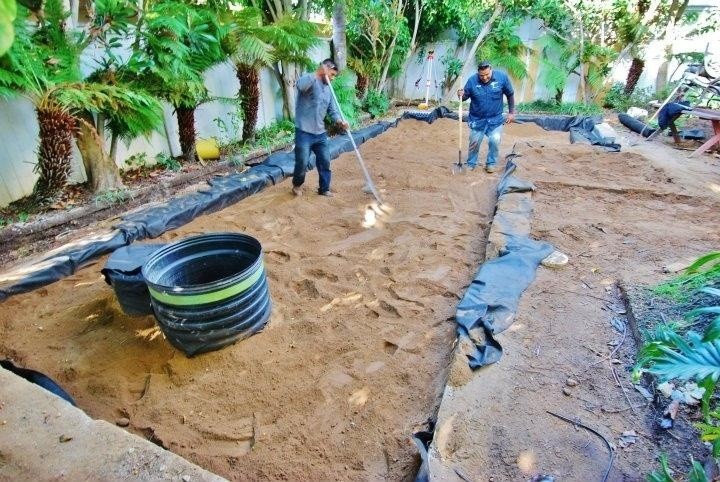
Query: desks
646,104,720,158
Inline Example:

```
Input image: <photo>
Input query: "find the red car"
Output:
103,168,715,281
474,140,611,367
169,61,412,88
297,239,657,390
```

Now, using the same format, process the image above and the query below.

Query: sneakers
322,191,334,196
486,164,495,173
292,179,302,196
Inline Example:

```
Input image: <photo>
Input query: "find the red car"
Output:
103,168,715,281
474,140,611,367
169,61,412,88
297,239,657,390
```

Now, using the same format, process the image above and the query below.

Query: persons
291,58,349,199
456,59,517,173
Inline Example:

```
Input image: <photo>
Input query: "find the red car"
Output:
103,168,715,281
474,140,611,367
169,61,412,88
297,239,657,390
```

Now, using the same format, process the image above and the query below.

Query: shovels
320,61,386,206
450,76,468,176
628,72,691,147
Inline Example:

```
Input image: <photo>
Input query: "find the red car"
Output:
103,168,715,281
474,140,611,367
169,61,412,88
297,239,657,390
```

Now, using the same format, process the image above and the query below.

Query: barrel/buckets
141,232,272,357
193,136,221,162
